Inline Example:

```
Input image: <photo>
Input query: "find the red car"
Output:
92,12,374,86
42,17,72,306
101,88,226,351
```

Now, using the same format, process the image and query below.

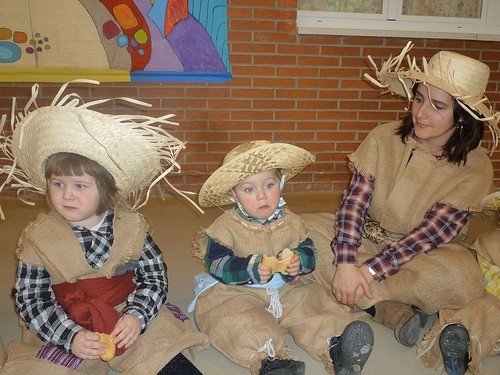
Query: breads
262,254,292,275
94,331,116,362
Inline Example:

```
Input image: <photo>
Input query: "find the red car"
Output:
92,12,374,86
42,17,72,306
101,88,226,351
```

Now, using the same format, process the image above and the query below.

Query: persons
296,39,500,375
0,79,210,375
187,139,374,375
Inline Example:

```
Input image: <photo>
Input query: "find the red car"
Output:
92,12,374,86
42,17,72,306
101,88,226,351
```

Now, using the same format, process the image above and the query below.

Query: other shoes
394,308,429,348
329,320,375,375
439,322,470,375
258,357,305,375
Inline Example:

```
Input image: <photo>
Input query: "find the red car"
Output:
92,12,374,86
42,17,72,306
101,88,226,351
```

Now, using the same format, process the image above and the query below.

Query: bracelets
367,267,383,283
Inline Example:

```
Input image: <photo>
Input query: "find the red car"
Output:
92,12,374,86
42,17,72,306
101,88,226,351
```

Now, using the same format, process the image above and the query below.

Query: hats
0,78,204,220
364,38,500,158
198,139,316,208
